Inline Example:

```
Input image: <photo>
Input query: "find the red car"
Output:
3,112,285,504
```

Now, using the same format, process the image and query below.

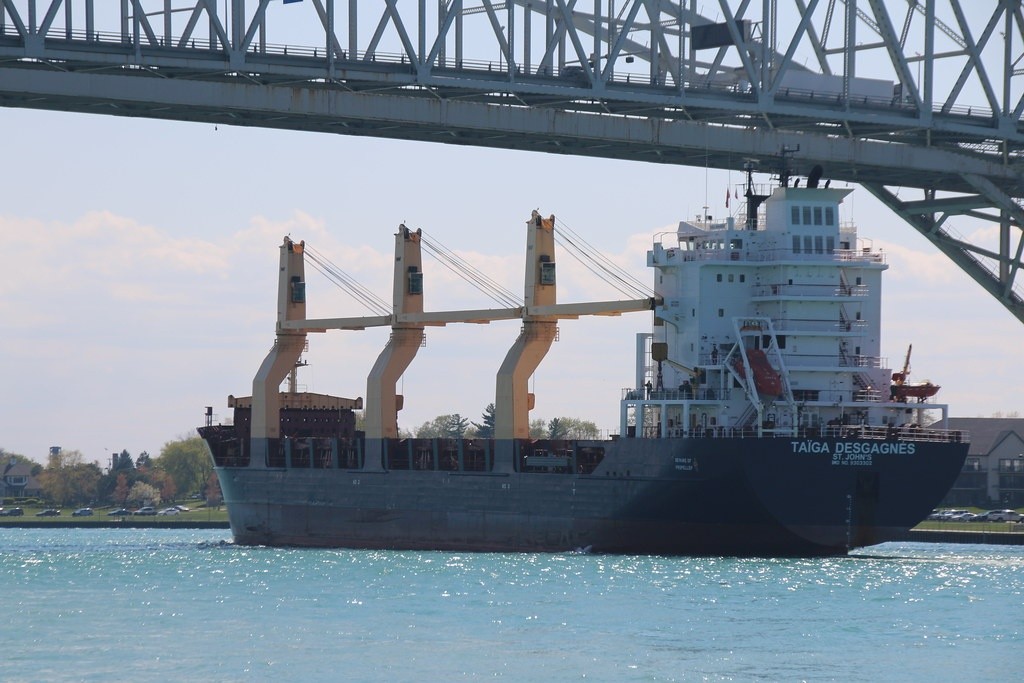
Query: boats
198,142,972,552
737,349,782,396
891,382,940,398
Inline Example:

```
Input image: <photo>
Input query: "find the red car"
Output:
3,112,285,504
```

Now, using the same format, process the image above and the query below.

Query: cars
134,507,157,515
72,508,93,516
158,508,180,516
3,508,24,516
929,510,991,522
36,510,60,517
192,494,200,499
174,505,190,512
107,510,132,516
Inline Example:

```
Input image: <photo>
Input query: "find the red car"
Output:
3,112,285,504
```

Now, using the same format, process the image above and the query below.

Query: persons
711,346,718,365
644,381,652,400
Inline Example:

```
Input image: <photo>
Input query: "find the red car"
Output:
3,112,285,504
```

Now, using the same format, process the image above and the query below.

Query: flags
726,188,730,208
735,188,738,199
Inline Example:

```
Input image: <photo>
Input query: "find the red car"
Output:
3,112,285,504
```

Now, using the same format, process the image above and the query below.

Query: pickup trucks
987,510,1024,523
